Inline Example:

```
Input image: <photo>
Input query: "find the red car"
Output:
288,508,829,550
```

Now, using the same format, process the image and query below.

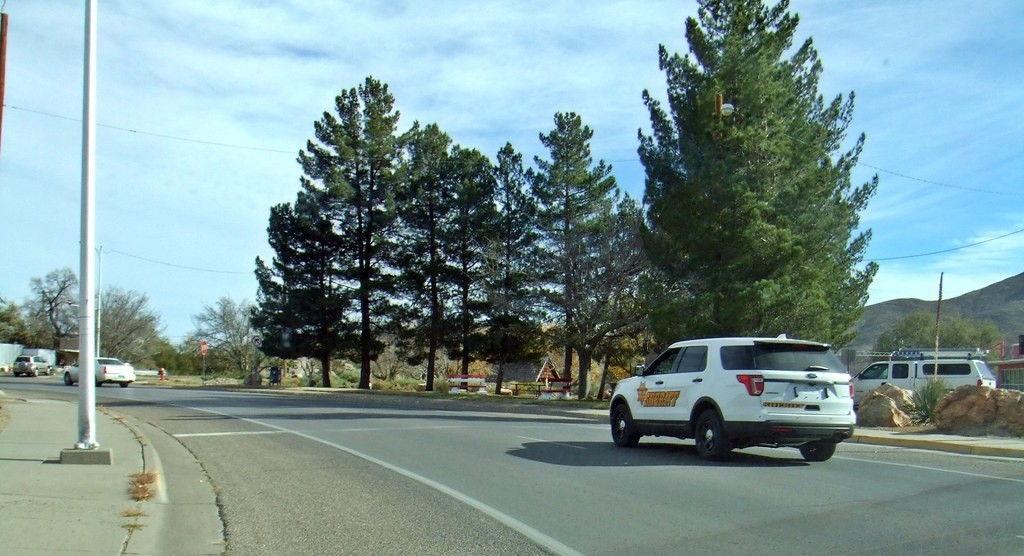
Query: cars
61,356,136,387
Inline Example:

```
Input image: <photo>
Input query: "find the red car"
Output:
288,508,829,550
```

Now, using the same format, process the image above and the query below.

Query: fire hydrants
160,368,167,381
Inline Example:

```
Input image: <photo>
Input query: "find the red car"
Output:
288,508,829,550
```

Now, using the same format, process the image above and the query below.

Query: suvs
609,334,858,462
13,354,55,377
852,358,997,409
